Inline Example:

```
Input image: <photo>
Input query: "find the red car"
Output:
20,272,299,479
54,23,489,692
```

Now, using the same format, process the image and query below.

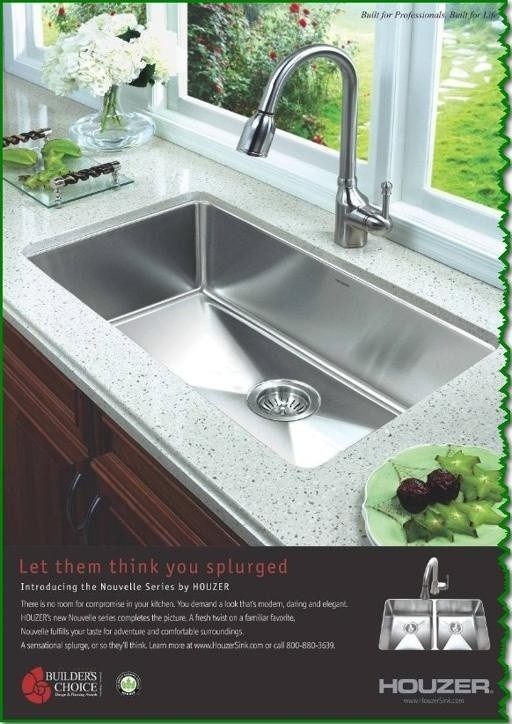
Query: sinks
22,191,503,469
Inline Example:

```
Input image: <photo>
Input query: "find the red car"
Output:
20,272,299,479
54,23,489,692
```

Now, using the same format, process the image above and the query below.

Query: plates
359,443,504,546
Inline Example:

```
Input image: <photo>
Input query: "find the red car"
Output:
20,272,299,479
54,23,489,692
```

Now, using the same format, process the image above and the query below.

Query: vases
70,86,156,154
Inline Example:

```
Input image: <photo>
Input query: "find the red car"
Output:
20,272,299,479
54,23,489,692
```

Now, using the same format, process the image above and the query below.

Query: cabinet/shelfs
0,323,249,548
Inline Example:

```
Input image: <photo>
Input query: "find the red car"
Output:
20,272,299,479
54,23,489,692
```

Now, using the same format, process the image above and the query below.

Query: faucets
235,44,394,251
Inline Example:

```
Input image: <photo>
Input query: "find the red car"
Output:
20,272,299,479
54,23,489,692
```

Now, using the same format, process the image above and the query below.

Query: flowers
42,14,170,135
